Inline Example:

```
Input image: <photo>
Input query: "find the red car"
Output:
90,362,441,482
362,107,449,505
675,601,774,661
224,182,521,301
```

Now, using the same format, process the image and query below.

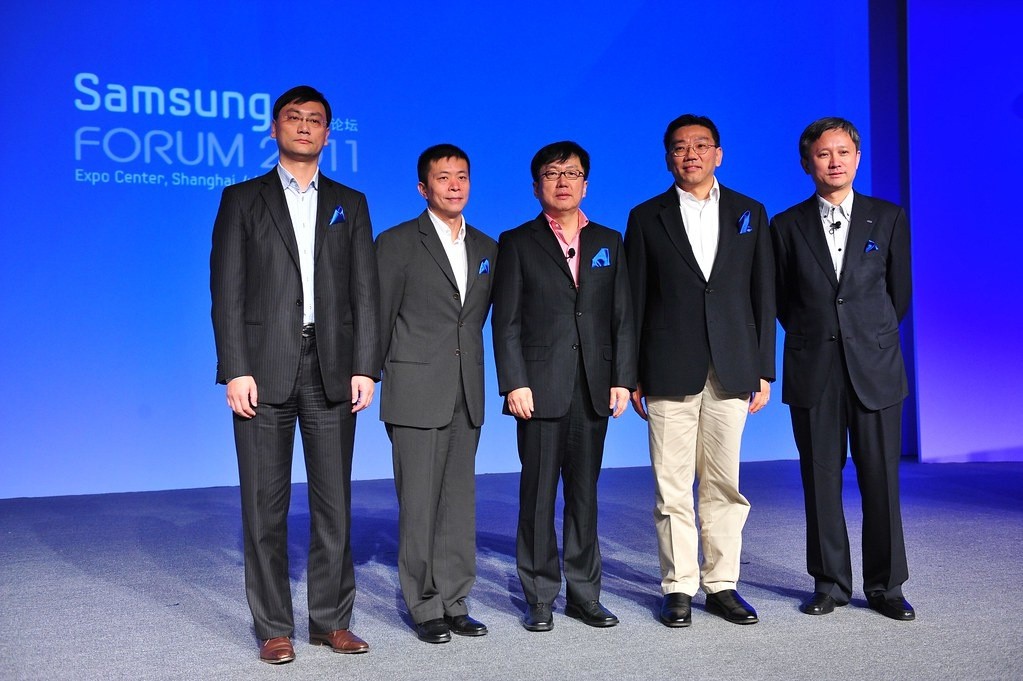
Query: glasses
537,169,584,180
668,142,716,157
277,112,328,126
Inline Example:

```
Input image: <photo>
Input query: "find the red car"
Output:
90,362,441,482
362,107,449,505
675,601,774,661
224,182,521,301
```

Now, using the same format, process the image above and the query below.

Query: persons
768,117,915,620
375,144,500,643
491,141,637,631
624,114,776,627
209,85,380,664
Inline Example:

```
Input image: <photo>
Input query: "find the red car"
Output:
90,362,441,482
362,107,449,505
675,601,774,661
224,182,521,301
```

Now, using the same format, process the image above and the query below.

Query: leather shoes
259,636,295,663
803,592,848,614
867,596,915,620
705,590,758,624
660,592,691,627
309,629,368,653
565,600,619,627
523,604,554,631
417,618,450,643
444,614,488,636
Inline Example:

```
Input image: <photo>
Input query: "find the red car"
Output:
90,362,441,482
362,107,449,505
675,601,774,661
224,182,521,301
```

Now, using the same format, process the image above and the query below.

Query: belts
303,324,316,337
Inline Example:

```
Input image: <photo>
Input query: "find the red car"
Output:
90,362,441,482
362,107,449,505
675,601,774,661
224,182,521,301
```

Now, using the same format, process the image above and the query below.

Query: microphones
832,221,841,229
569,248,575,259
